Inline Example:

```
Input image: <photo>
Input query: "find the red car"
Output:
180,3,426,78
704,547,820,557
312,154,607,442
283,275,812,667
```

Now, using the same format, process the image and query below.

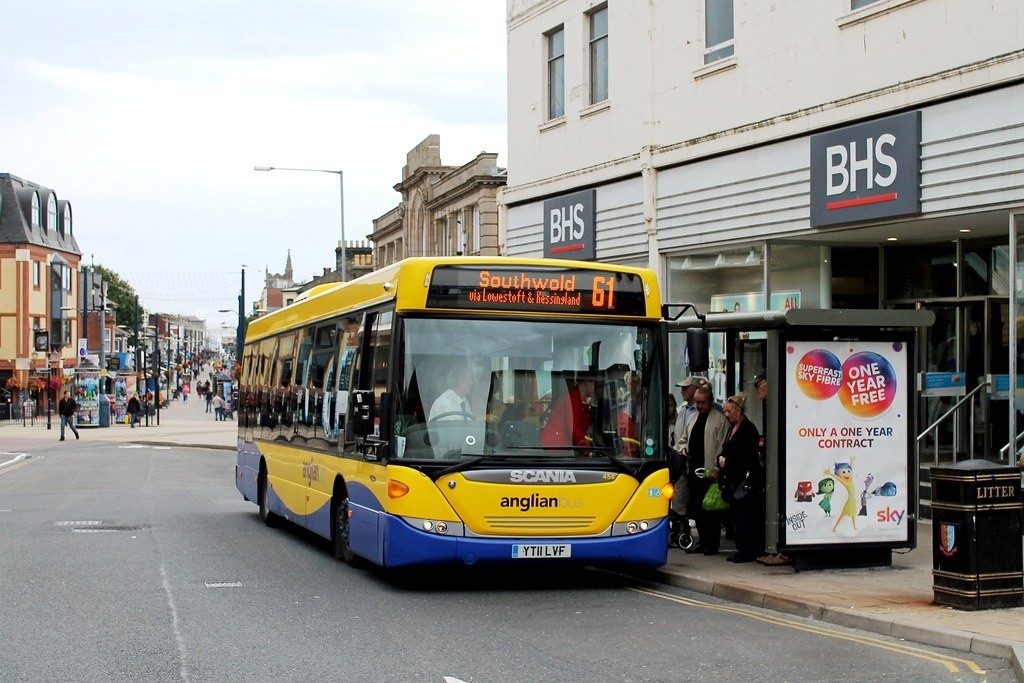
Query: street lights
220,309,268,339
60,308,112,362
92,283,100,310
254,165,347,282
117,325,201,427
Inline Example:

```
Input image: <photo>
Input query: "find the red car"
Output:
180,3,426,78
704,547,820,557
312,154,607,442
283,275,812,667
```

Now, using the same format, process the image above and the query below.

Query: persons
209,370,213,380
213,392,233,421
127,392,140,428
206,388,212,413
183,385,190,404
541,366,596,458
196,379,210,399
616,370,791,566
427,366,507,423
59,390,79,441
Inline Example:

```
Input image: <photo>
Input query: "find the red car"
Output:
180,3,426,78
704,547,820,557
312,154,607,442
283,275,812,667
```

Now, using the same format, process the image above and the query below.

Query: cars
0,387,11,404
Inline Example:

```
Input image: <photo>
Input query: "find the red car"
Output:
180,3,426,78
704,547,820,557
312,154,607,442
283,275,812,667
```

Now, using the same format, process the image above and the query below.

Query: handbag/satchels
695,467,730,511
670,475,698,516
743,467,784,507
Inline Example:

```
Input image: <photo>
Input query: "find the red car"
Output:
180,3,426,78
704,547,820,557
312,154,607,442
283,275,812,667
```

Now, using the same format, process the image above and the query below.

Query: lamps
714,252,725,266
680,258,692,269
745,247,759,264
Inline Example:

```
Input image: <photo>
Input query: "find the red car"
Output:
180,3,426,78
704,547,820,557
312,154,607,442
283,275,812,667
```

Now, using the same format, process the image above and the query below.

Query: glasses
725,396,741,408
754,380,765,388
682,386,689,391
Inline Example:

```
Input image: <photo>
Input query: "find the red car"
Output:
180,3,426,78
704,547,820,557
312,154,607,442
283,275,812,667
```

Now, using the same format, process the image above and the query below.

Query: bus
235,255,710,571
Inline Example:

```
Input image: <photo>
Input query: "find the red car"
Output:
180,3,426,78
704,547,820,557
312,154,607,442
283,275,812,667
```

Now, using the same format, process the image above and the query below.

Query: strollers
666,468,709,551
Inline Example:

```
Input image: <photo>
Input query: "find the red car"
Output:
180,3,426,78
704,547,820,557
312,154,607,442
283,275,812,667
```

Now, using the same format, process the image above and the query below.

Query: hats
747,369,767,384
675,377,697,387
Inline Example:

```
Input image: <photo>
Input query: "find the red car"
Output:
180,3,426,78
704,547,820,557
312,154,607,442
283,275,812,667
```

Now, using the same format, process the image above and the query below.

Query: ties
460,401,467,420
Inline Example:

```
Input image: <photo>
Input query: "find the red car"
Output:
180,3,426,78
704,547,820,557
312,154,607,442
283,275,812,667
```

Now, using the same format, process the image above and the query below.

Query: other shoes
704,545,719,555
726,549,758,562
683,542,705,553
764,552,793,566
756,554,775,563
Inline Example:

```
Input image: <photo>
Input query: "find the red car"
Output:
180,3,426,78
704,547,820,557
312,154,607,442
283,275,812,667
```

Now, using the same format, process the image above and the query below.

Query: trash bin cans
929,459,1024,611
233,392,239,410
